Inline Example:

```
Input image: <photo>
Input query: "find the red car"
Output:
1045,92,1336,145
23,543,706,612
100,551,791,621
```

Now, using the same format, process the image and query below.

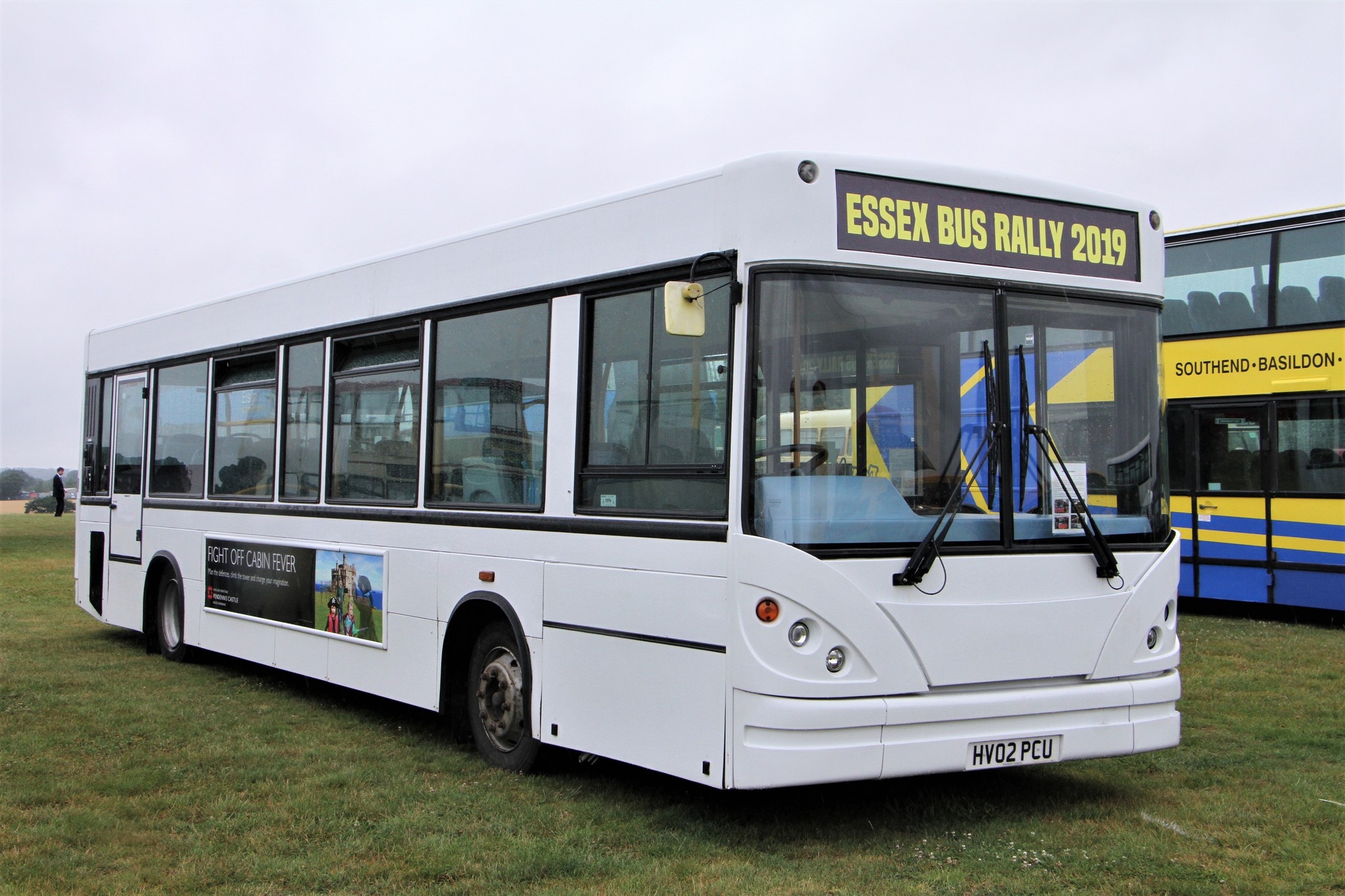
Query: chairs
1160,273,1345,335
164,435,726,527
1170,443,1345,493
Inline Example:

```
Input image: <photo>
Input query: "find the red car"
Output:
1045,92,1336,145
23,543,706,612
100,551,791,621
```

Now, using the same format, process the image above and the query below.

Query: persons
219,456,266,495
53,467,68,517
324,575,380,643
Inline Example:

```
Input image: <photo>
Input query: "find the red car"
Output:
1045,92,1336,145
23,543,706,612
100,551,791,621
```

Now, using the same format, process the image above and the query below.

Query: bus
850,204,1344,630
755,408,852,464
73,151,1184,794
